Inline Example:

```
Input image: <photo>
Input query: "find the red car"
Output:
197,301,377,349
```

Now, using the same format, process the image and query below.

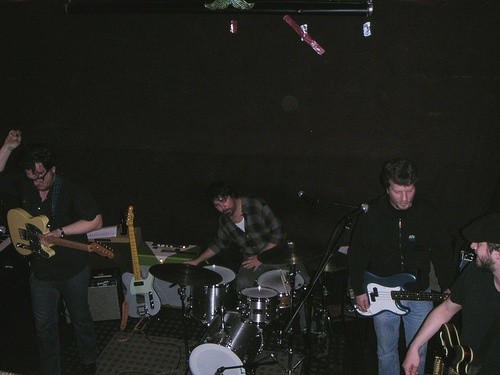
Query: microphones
217,366,226,373
297,191,319,203
339,203,368,223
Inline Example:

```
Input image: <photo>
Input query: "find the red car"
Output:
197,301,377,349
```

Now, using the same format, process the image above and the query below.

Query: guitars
7,207,115,259
347,270,453,316
434,321,473,375
121,203,161,319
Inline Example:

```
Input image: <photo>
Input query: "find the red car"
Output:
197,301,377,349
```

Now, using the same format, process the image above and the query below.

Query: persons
182,181,317,335
402,212,500,375
347,157,457,375
0,150,104,375
0,129,22,172
86,202,142,308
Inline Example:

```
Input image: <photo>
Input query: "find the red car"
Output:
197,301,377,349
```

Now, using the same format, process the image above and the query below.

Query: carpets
95,331,302,375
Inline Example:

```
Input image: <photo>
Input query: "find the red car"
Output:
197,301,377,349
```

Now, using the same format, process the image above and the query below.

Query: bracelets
59,227,65,239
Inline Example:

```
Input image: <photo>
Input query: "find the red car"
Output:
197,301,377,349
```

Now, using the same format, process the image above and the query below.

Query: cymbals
257,244,326,265
305,250,350,272
149,263,222,286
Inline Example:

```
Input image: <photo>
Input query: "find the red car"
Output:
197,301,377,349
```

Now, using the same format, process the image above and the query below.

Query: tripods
289,297,331,375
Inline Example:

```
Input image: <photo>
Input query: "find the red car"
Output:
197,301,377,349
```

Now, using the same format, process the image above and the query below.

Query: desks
140,245,201,307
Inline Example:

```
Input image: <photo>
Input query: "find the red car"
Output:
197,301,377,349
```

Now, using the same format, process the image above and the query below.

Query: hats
462,213,500,244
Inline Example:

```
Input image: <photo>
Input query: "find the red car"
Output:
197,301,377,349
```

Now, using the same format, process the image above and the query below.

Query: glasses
27,168,49,184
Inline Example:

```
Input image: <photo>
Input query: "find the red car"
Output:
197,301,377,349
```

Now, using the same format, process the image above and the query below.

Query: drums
256,270,306,309
186,311,263,375
237,287,281,326
189,266,237,323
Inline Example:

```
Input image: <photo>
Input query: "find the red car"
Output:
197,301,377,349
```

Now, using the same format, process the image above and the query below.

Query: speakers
61,267,124,326
